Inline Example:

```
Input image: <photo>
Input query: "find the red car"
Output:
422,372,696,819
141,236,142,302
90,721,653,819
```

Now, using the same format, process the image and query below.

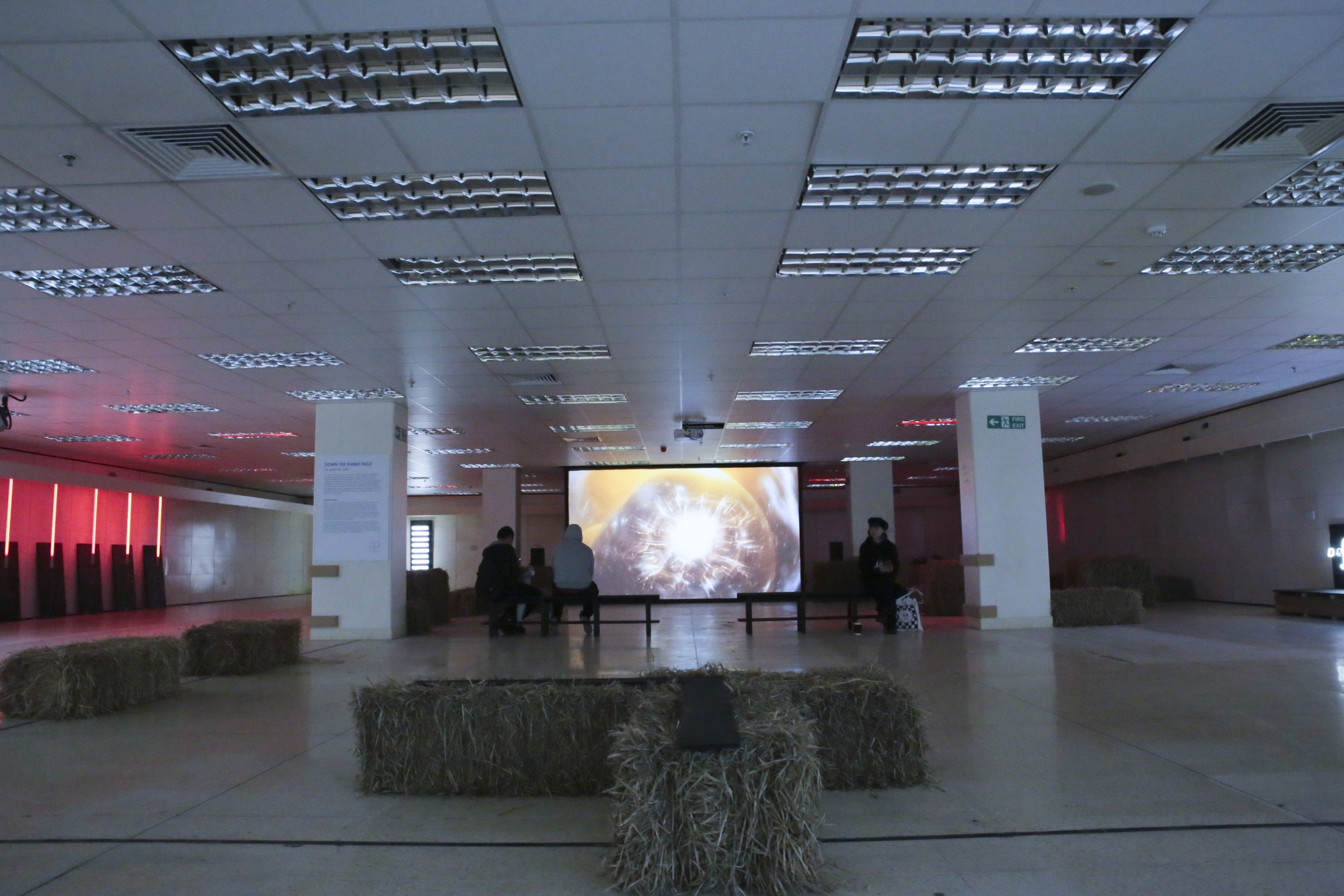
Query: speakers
530,548,545,566
830,541,845,562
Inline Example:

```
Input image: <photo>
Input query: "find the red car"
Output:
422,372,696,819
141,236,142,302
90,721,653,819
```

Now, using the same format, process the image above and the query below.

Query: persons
858,517,902,635
552,524,598,636
475,525,528,635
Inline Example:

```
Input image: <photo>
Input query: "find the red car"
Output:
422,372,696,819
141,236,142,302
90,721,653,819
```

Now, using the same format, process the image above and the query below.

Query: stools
183,618,323,676
0,632,188,718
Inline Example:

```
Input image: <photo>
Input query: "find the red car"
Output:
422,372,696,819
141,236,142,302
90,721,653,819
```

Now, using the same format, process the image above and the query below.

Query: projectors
674,429,703,442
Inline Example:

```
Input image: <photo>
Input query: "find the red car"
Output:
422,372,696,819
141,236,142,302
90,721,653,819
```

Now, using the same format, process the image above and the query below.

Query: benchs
1275,586,1342,622
735,590,884,633
484,590,660,636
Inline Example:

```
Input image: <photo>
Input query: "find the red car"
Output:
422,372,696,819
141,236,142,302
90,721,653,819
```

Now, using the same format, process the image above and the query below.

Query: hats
868,517,888,530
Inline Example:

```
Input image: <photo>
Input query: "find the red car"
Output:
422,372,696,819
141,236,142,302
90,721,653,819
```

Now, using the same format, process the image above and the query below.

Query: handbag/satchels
896,588,924,631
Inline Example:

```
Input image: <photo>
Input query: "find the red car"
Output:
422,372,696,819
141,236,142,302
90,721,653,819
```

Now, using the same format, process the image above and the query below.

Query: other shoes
581,616,593,634
550,616,560,634
515,622,526,634
